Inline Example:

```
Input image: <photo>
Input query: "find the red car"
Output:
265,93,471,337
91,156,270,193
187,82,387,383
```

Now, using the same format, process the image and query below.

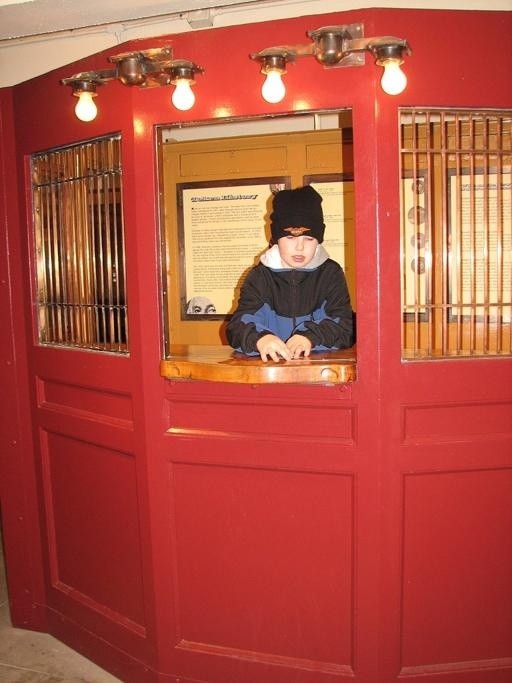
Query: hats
269,186,326,244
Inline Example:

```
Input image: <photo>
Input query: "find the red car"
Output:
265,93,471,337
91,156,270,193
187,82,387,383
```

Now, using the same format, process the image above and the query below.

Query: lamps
58,41,204,124
249,23,413,106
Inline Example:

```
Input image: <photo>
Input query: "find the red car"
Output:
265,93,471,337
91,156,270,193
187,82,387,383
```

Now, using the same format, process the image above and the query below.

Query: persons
225,185,355,363
189,295,217,315
